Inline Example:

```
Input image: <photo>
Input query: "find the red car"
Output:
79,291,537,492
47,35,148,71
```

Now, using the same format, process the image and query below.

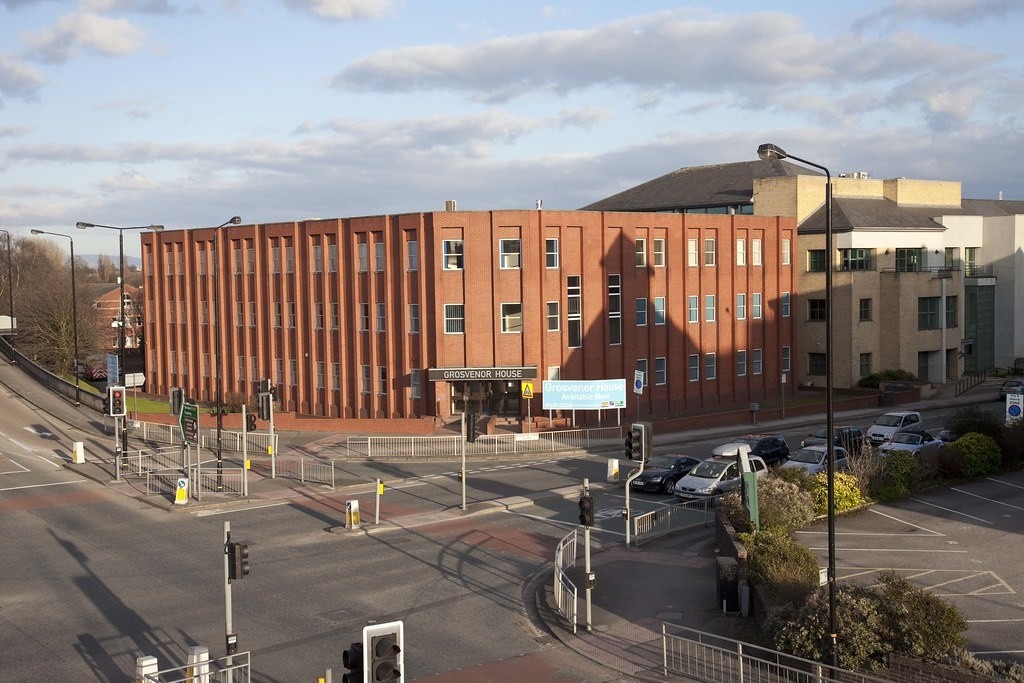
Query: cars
936,427,963,444
800,426,865,453
626,453,708,496
999,379,1024,398
671,443,769,507
865,411,927,443
780,445,853,476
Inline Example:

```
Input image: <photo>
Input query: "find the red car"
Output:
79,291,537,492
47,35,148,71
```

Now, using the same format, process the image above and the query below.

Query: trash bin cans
715,556,739,613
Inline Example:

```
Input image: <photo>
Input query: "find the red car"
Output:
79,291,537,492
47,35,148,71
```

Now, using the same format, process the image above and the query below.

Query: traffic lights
588,572,597,589
458,472,462,482
269,387,279,403
579,498,595,528
363,621,407,683
625,438,631,457
227,543,250,581
109,386,129,417
622,507,628,521
102,397,111,417
227,634,238,654
246,414,257,434
116,447,121,454
259,379,271,394
627,423,645,464
342,643,370,683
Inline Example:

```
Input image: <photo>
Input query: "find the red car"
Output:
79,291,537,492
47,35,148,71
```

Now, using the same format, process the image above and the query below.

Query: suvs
728,433,791,470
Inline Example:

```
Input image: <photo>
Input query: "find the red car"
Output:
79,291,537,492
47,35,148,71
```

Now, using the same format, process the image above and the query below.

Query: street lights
214,216,247,487
30,228,83,409
760,142,842,680
76,221,168,471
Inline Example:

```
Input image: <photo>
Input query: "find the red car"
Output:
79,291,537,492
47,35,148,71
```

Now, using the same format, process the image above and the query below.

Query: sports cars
876,431,946,459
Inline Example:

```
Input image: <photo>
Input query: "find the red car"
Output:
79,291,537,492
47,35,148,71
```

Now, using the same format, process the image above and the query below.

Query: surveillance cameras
112,323,118,330
